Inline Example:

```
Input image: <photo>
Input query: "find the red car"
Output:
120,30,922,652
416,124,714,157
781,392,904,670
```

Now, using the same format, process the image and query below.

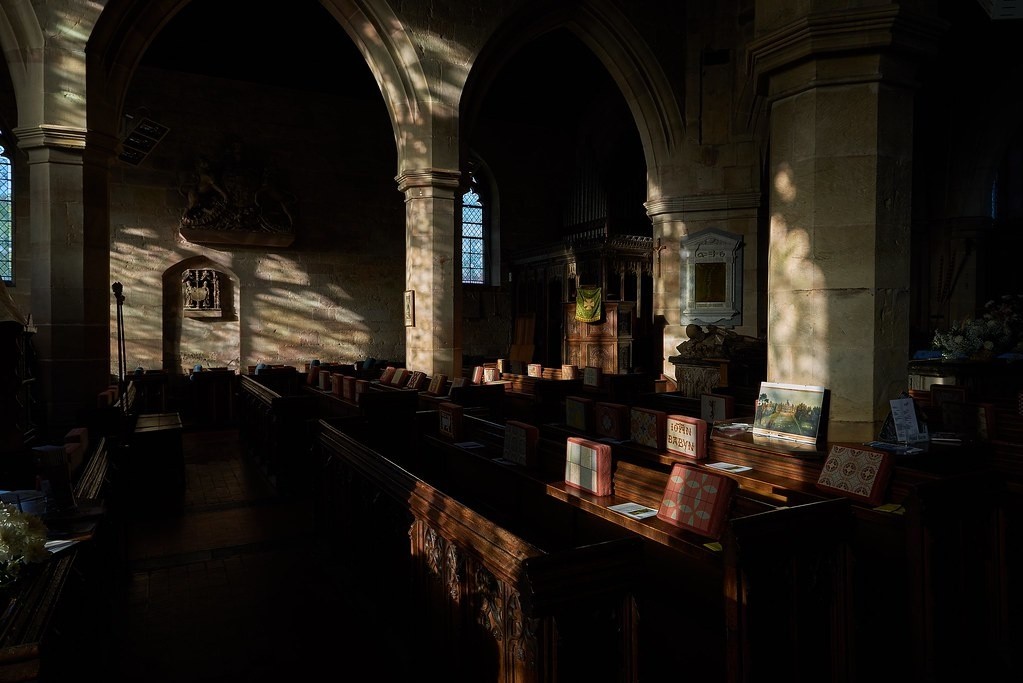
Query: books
931,432,962,441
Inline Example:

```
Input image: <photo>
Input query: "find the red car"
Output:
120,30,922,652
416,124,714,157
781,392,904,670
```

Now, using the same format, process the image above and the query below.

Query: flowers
932,295,1023,362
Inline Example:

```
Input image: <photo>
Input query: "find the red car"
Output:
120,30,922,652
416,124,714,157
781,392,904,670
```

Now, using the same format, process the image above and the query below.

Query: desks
0,380,184,683
188,367,1023,683
